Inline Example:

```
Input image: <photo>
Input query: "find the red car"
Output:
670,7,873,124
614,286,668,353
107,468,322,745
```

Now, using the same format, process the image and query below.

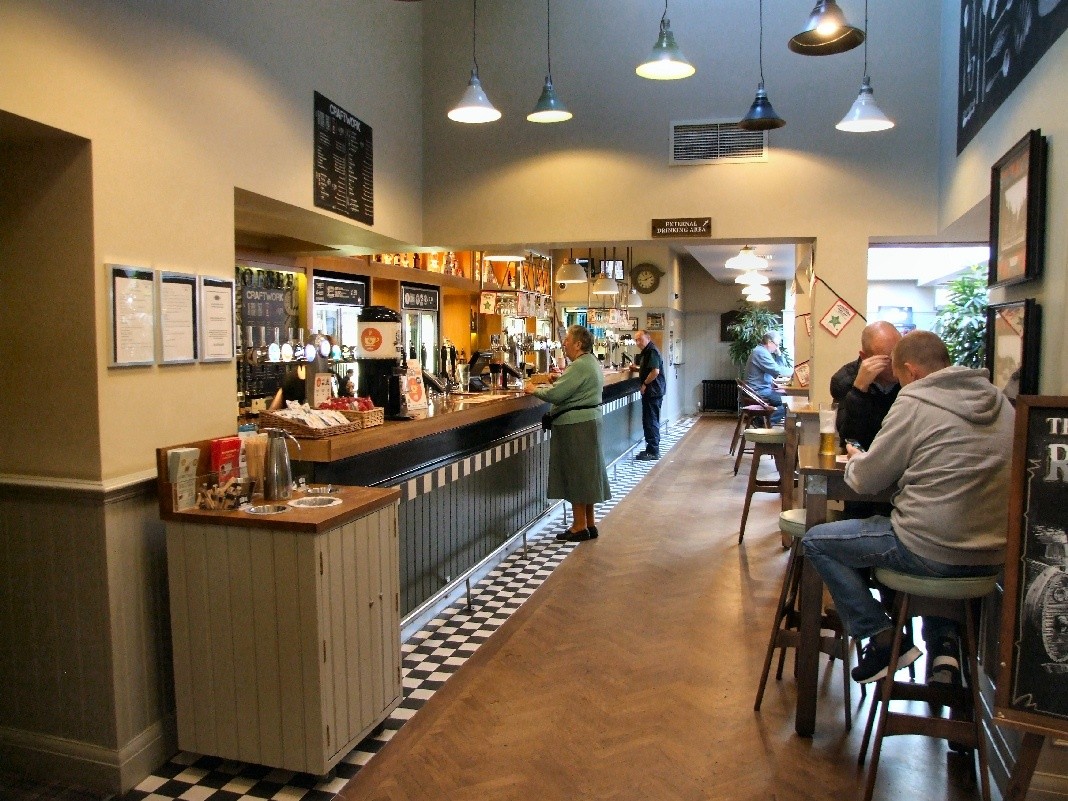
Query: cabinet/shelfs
171,502,405,777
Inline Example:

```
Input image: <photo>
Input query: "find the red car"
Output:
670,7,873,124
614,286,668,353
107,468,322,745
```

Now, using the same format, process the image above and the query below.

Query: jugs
237,423,257,477
263,427,302,501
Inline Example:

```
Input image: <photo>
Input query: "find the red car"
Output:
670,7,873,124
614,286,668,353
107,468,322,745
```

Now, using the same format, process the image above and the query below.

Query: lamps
789,1,864,57
526,0,573,124
592,247,619,294
619,247,643,308
724,244,772,303
482,248,527,262
835,2,892,133
636,1,697,81
447,0,503,124
738,0,785,133
555,248,587,283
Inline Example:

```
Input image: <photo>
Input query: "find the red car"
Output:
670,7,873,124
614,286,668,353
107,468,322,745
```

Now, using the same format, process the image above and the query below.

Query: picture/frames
985,297,1037,406
988,128,1046,290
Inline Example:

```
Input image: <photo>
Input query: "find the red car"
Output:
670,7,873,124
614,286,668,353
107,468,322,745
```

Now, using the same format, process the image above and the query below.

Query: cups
819,402,839,455
245,433,268,494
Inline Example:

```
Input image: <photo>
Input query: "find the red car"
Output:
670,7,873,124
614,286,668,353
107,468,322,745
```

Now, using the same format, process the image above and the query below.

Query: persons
629,330,666,461
829,322,913,631
522,325,612,542
742,333,794,428
799,331,1014,688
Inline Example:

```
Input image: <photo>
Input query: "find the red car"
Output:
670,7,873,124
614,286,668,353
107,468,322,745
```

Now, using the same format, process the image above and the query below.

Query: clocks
630,262,661,294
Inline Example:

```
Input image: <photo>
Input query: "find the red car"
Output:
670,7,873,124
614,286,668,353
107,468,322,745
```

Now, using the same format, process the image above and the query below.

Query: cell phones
845,438,866,454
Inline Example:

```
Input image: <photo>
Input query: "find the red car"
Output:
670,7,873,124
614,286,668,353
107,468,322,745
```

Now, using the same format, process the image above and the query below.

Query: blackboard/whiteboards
992,393,1068,744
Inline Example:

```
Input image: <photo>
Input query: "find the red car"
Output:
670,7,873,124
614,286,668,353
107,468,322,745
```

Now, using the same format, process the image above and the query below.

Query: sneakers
640,451,661,458
589,526,598,538
636,452,659,460
556,528,590,541
852,633,922,683
925,643,960,686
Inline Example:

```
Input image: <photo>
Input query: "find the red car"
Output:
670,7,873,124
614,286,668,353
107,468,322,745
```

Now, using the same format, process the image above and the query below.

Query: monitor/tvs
465,351,490,391
599,260,625,281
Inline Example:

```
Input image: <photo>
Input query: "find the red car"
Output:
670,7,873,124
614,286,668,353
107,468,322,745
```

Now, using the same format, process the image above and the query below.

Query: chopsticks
196,477,242,511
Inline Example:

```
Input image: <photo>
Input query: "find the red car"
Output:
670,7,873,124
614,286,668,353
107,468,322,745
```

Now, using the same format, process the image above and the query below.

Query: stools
858,567,998,801
728,378,915,736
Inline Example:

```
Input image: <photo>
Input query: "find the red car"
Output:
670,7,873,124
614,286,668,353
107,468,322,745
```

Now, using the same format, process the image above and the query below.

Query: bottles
375,253,420,269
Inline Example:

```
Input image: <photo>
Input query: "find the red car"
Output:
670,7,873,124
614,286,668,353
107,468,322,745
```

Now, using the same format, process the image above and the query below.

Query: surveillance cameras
559,282,567,291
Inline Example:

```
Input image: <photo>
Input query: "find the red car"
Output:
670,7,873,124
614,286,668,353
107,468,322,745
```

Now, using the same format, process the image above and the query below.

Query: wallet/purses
542,411,551,432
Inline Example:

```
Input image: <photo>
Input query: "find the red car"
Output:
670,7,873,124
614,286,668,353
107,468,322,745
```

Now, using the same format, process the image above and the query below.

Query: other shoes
752,417,763,428
